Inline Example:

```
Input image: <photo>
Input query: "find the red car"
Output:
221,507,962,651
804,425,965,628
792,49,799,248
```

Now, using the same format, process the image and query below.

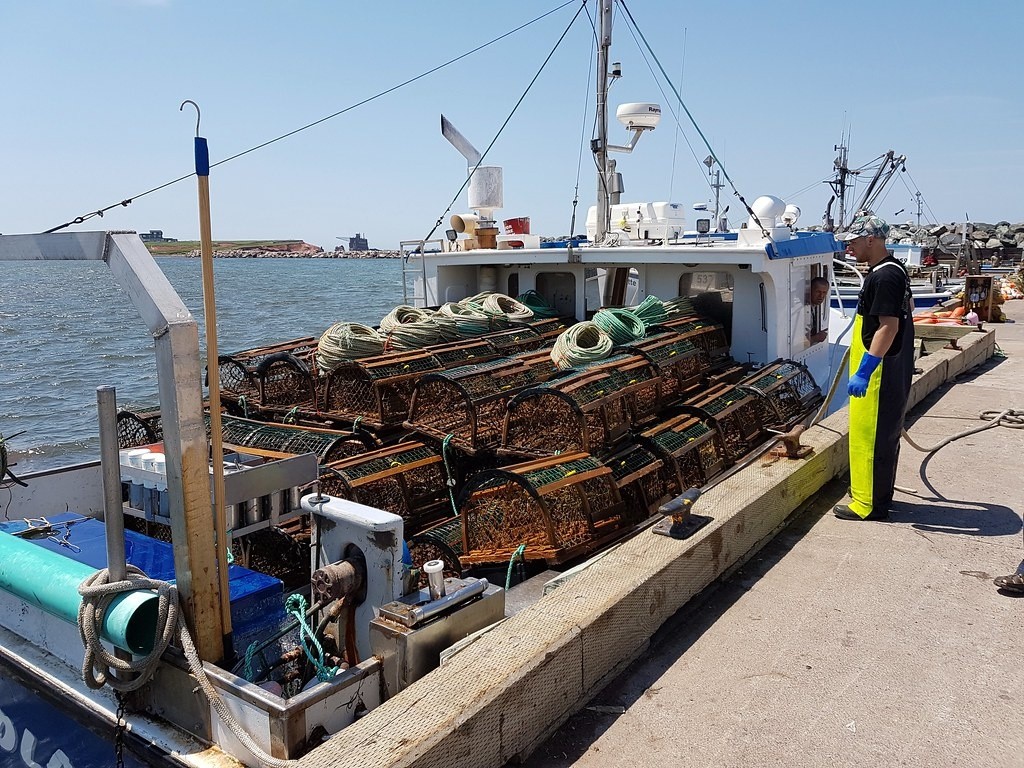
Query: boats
1,2,1018,767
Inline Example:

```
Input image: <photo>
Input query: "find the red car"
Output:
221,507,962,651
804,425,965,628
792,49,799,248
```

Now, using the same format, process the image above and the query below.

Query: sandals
994,574,1024,592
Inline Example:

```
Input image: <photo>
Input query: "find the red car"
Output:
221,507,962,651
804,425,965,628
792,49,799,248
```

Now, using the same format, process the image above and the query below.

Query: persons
811,277,830,344
922,251,939,267
832,215,915,522
990,252,1002,268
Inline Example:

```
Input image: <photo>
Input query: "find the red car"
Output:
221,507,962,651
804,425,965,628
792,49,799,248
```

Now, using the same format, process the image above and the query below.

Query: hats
834,216,890,242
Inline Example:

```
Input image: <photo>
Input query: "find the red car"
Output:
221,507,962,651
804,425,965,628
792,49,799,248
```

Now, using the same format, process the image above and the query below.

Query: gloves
847,352,882,398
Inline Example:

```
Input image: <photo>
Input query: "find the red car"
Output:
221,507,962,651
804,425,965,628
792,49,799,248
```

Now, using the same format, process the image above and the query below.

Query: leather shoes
841,481,852,497
832,504,862,519
